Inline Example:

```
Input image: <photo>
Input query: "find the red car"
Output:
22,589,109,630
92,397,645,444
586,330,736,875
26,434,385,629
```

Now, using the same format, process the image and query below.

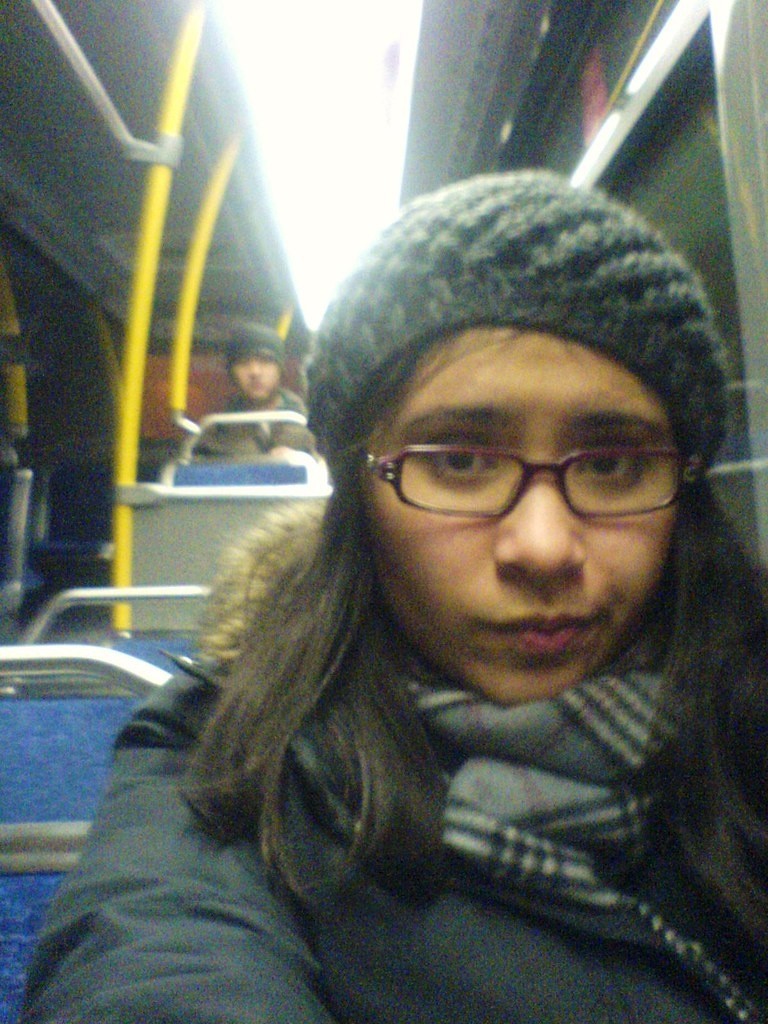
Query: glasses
355,446,702,518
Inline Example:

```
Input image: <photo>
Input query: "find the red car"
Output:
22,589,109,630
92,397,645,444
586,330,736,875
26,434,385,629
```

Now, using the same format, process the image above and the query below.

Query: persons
200,323,315,460
19,167,767,1024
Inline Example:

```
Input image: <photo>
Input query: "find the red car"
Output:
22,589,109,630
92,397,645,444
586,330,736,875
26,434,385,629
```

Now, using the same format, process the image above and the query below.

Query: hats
226,322,284,372
305,168,730,497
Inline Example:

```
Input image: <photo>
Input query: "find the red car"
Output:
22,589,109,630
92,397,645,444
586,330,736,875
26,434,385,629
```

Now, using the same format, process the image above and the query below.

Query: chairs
163,407,331,493
0,584,223,1024
